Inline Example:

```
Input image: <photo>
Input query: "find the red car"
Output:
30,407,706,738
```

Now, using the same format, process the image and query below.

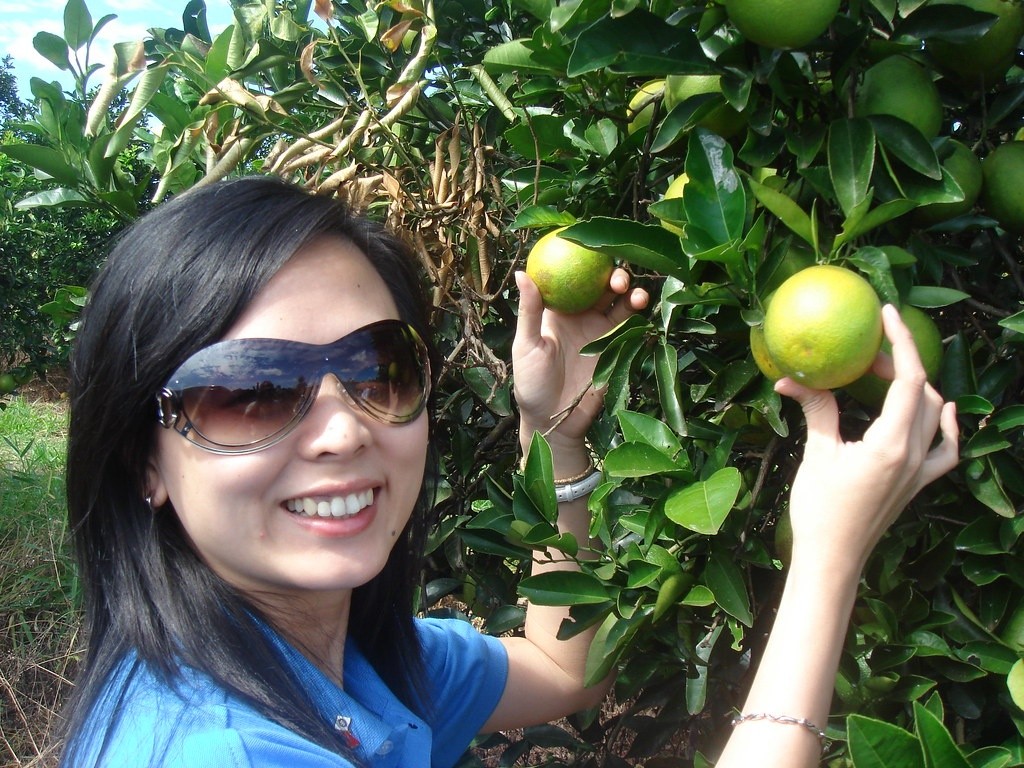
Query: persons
58,176,958,768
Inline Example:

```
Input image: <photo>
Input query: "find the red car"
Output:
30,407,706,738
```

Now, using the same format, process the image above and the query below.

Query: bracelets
519,453,593,484
730,714,832,760
555,471,602,503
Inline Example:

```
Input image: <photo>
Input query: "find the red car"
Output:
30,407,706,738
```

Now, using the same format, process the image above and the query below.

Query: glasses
154,319,434,455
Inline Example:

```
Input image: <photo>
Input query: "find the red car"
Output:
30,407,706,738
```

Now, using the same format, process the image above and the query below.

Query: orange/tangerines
525,0,1024,651
